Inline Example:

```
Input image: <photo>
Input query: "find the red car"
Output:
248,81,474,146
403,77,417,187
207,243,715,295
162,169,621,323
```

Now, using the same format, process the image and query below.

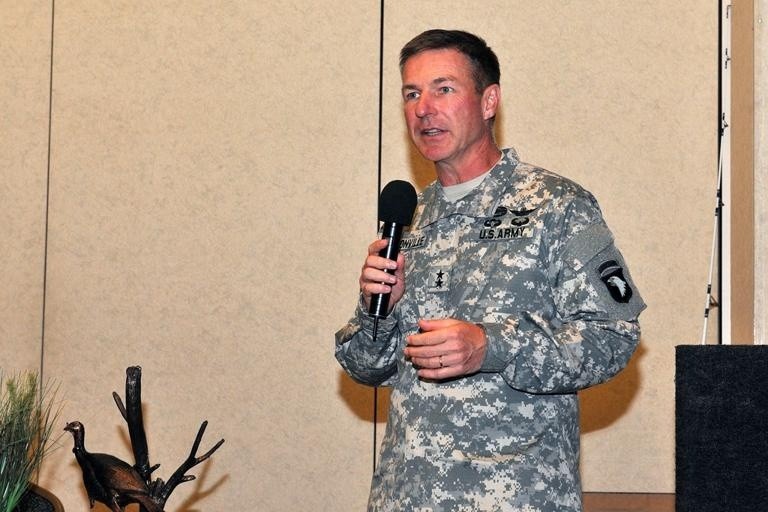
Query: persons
332,29,648,511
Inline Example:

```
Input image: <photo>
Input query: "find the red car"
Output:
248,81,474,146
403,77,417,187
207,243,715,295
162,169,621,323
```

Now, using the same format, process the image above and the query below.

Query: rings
438,356,443,368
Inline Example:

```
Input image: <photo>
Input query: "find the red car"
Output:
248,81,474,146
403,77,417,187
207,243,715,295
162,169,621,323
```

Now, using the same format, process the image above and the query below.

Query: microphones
369,180,418,341
